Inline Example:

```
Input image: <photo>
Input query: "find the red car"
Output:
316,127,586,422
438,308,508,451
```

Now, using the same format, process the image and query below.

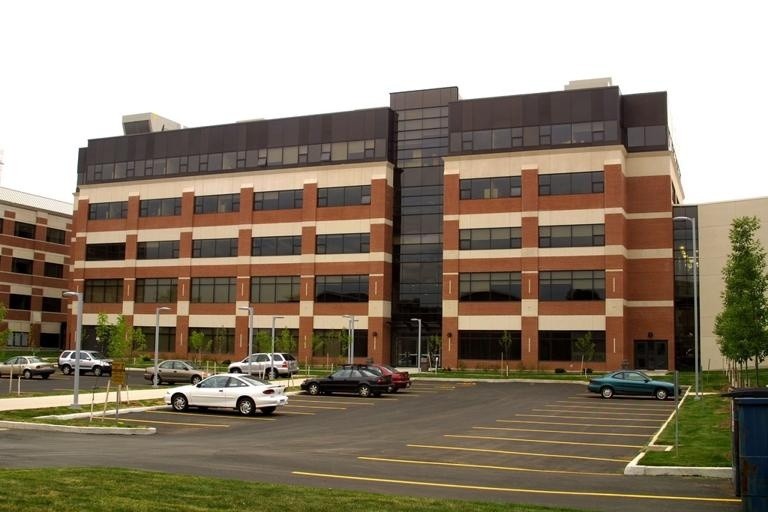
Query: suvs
227,351,299,379
58,349,116,376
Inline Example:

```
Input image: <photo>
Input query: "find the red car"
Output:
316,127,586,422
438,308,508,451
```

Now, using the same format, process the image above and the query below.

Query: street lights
343,313,361,366
236,306,255,381
270,314,287,380
59,290,86,408
410,318,423,373
151,304,175,388
670,209,704,402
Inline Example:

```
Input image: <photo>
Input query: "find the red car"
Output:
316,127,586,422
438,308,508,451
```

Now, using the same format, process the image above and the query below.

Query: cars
159,371,294,419
0,355,56,380
587,366,687,406
398,351,440,367
143,359,214,386
340,363,413,394
298,365,395,399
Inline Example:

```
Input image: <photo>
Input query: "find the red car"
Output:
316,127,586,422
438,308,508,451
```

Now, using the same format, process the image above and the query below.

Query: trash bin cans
719,391,768,512
420,357,429,371
